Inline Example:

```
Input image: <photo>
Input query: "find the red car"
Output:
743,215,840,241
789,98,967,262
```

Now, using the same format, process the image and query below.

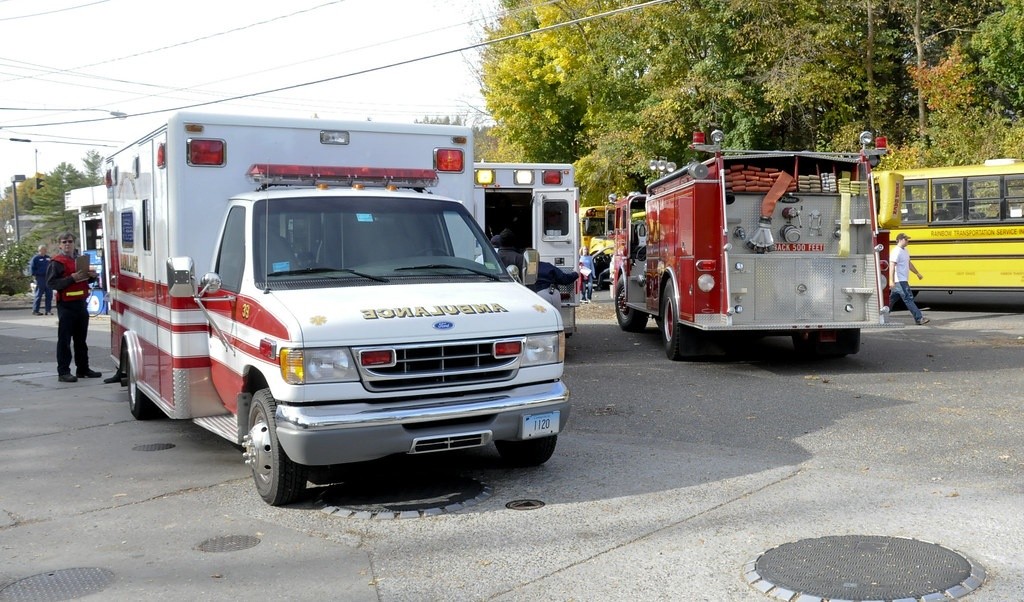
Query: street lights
10,174,26,279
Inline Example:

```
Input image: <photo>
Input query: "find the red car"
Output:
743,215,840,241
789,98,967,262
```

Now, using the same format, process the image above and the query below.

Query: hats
897,233,911,239
491,235,501,245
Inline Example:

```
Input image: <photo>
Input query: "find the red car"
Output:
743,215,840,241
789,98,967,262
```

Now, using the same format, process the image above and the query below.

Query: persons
30,245,54,316
579,246,596,304
46,232,102,382
889,234,929,325
947,186,963,220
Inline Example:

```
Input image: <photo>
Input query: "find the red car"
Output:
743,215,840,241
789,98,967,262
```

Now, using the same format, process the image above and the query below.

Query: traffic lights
35,172,45,190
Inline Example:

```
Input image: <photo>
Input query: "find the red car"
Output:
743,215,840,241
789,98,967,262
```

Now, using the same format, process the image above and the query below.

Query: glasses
60,240,74,244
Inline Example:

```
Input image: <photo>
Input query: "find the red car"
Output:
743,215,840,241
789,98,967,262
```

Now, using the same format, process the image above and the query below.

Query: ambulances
469,161,582,341
103,111,573,506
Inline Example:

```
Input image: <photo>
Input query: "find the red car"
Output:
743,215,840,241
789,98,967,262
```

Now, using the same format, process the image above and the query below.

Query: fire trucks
606,129,889,368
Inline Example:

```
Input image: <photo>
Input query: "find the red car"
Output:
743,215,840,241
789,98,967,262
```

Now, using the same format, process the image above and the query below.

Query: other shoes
45,311,53,315
32,310,43,315
104,374,127,383
588,299,591,303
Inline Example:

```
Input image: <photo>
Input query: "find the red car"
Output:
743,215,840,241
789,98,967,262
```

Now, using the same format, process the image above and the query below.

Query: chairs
901,208,987,221
254,231,297,276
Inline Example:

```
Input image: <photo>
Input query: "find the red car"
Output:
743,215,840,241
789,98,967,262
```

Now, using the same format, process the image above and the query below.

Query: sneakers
59,372,77,382
916,318,929,325
76,368,102,378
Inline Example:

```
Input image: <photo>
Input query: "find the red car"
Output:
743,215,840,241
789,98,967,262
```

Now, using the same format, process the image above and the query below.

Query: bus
580,206,613,265
838,158,1024,307
